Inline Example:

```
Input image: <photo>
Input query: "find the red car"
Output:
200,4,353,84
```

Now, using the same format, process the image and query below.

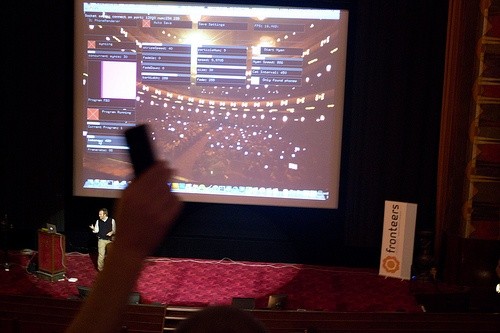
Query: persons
68,158,263,333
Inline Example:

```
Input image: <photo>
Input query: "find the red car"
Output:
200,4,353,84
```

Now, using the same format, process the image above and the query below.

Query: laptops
47,224,56,233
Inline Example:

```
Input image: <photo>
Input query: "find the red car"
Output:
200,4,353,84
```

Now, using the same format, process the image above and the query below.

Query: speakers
127,292,141,304
78,286,91,297
232,297,255,309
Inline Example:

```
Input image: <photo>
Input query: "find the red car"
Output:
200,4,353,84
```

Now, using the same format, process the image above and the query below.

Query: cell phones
125,125,157,180
